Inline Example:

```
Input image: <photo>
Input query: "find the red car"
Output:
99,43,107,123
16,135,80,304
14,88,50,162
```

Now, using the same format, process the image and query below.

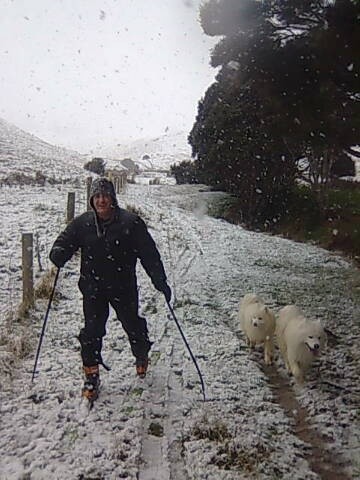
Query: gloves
153,281,171,303
49,249,68,267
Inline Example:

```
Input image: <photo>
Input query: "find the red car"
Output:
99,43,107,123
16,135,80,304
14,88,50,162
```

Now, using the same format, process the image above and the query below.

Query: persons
48,176,171,401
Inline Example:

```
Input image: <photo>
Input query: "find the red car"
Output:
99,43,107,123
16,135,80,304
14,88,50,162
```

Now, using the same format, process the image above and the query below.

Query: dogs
239,293,276,366
276,304,327,383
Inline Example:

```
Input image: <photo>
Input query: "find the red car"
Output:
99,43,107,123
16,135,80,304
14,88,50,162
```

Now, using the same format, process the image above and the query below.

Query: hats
89,178,120,237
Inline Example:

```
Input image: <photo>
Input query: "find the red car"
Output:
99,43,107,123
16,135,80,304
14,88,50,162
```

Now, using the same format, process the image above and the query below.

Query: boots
82,364,101,401
136,353,149,378
